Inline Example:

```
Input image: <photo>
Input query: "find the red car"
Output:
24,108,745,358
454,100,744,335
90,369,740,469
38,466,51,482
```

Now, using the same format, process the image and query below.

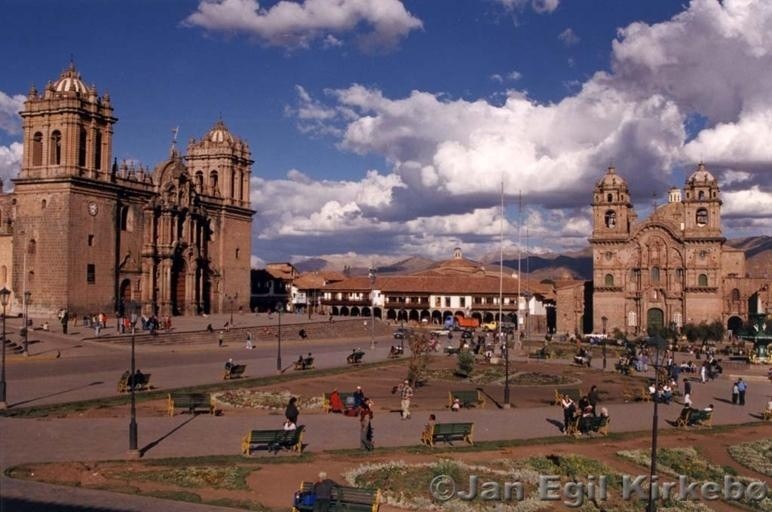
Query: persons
15,306,772,512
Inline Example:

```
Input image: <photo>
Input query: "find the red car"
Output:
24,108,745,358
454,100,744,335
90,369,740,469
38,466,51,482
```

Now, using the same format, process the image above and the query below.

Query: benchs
448,391,485,410
167,392,215,416
322,392,370,413
420,422,474,448
242,424,305,454
566,414,610,437
117,373,151,392
614,363,637,374
676,408,714,430
292,480,381,512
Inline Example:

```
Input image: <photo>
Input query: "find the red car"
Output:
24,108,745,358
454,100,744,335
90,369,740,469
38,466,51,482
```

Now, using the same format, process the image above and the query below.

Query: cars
584,329,608,344
392,328,409,339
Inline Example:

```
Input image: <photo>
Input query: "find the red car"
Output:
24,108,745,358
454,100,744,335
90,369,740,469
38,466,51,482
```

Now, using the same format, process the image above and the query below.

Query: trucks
443,315,479,331
480,321,497,332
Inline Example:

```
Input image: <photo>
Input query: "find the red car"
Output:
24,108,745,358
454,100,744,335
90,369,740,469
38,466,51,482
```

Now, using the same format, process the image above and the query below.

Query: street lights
124,299,141,459
0,286,32,410
275,302,284,376
504,315,513,404
601,316,608,368
644,328,668,512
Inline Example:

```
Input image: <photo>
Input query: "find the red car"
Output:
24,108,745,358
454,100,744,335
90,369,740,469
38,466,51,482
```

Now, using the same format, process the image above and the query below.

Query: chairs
347,352,365,363
292,358,313,369
224,364,246,378
